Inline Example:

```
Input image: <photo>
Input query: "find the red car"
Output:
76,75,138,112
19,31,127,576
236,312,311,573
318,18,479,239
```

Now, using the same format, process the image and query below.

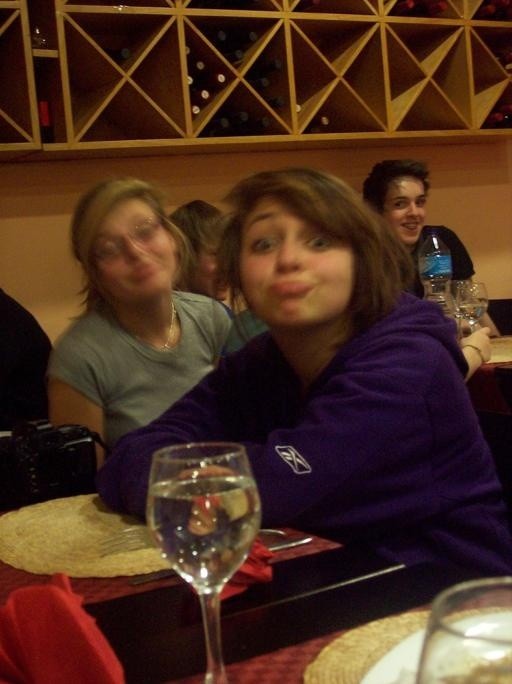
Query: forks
97,524,288,556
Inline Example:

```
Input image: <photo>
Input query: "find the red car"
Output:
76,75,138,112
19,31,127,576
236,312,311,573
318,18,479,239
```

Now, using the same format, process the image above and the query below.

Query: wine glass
146,441,264,683
446,280,488,339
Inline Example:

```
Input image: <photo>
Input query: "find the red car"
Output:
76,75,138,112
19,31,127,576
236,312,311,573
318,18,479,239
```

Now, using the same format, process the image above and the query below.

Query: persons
364,159,501,338
1,286,52,431
168,198,234,302
98,169,512,662
219,307,491,386
48,178,235,469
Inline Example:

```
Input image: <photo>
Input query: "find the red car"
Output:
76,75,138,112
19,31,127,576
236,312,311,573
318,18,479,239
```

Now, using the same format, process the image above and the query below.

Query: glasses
95,219,160,262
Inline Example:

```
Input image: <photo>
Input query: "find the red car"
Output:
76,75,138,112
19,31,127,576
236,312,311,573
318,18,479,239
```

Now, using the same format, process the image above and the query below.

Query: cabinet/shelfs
0,1,512,165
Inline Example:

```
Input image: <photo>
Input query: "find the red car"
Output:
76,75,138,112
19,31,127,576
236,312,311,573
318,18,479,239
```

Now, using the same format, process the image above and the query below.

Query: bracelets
462,344,485,365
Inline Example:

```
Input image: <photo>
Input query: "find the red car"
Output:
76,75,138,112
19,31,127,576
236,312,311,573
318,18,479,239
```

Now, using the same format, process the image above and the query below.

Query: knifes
129,536,313,585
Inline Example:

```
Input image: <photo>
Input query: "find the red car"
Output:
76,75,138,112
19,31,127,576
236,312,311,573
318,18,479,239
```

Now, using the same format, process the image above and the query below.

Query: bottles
296,105,302,113
295,0,320,11
103,47,130,64
32,23,48,48
312,116,329,128
185,30,285,137
495,52,512,65
485,104,511,130
37,100,54,143
392,0,512,19
417,228,454,305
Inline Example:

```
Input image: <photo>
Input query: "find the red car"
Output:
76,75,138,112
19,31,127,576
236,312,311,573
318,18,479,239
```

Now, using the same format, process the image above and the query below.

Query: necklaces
134,302,176,351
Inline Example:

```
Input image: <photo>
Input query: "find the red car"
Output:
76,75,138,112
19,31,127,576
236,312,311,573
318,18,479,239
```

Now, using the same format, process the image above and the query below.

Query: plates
362,611,512,684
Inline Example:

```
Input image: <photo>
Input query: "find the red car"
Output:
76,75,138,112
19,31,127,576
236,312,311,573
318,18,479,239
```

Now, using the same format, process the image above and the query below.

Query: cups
416,576,512,683
